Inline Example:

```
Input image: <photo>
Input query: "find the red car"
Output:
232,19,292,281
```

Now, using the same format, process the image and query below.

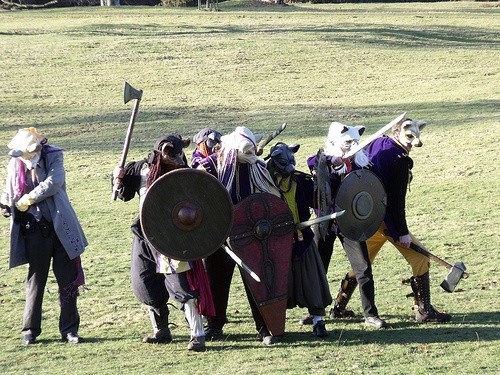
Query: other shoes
61,331,82,344
261,331,278,345
22,335,37,345
187,336,205,350
204,327,223,341
142,331,173,345
312,320,328,338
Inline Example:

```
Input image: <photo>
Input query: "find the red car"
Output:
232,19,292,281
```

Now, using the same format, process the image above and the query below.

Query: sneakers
414,305,452,324
364,316,387,328
329,308,355,319
299,314,314,325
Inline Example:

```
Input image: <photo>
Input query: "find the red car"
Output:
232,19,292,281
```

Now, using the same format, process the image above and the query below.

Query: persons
204,126,294,344
0,127,90,345
298,122,389,328
263,142,333,337
111,133,216,352
189,128,223,179
330,118,451,323
